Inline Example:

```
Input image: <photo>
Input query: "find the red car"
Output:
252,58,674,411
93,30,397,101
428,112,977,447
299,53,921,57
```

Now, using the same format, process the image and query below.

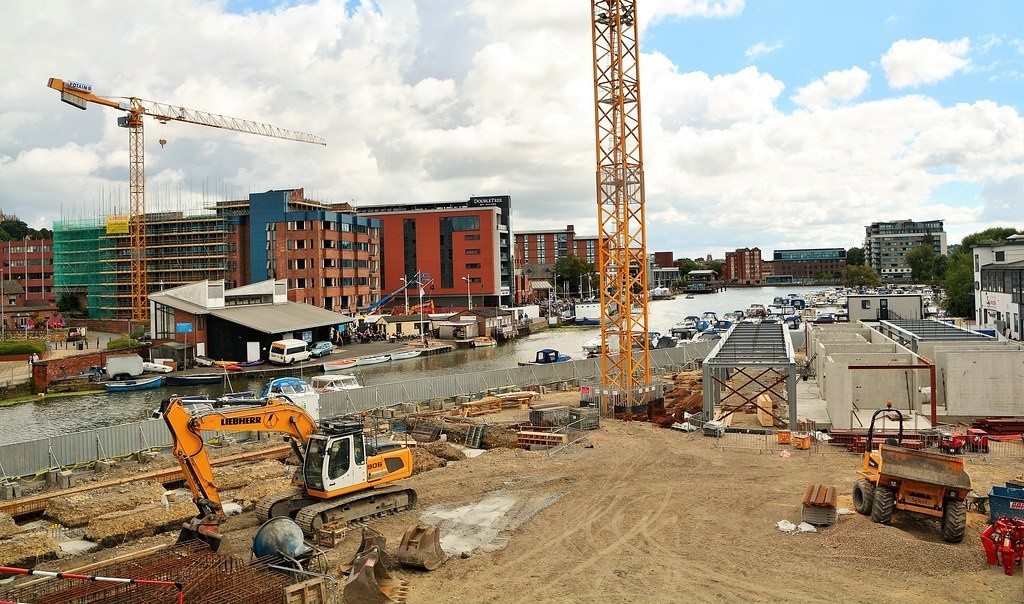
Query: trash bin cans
76,341,83,350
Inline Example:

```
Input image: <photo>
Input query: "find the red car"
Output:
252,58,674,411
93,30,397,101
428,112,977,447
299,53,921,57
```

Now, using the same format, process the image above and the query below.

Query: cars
308,341,334,358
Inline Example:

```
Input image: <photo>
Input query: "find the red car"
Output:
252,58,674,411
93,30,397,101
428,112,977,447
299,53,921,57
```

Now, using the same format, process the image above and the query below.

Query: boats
670,282,950,342
619,331,679,351
356,356,391,366
454,335,497,349
651,286,677,301
322,361,357,371
166,372,224,386
518,348,572,367
105,372,163,392
582,342,621,359
392,351,423,361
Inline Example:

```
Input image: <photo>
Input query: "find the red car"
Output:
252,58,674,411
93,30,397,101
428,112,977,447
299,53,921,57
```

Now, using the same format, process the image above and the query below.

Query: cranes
47,78,327,333
590,0,654,419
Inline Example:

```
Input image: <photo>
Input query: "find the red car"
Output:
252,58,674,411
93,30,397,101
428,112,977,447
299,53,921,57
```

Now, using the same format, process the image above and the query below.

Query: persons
335,441,349,471
423,337,429,348
27,353,39,372
315,443,331,457
357,327,404,344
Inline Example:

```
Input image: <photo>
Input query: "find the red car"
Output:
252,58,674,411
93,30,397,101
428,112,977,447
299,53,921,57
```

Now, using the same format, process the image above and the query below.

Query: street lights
461,273,473,311
400,274,409,314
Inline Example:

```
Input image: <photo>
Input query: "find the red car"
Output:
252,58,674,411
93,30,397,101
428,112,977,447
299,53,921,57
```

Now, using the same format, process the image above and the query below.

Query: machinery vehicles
159,391,418,560
851,400,974,542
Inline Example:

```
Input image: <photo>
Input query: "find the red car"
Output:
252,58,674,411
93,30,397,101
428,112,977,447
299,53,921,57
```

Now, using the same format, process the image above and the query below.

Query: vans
268,339,312,366
